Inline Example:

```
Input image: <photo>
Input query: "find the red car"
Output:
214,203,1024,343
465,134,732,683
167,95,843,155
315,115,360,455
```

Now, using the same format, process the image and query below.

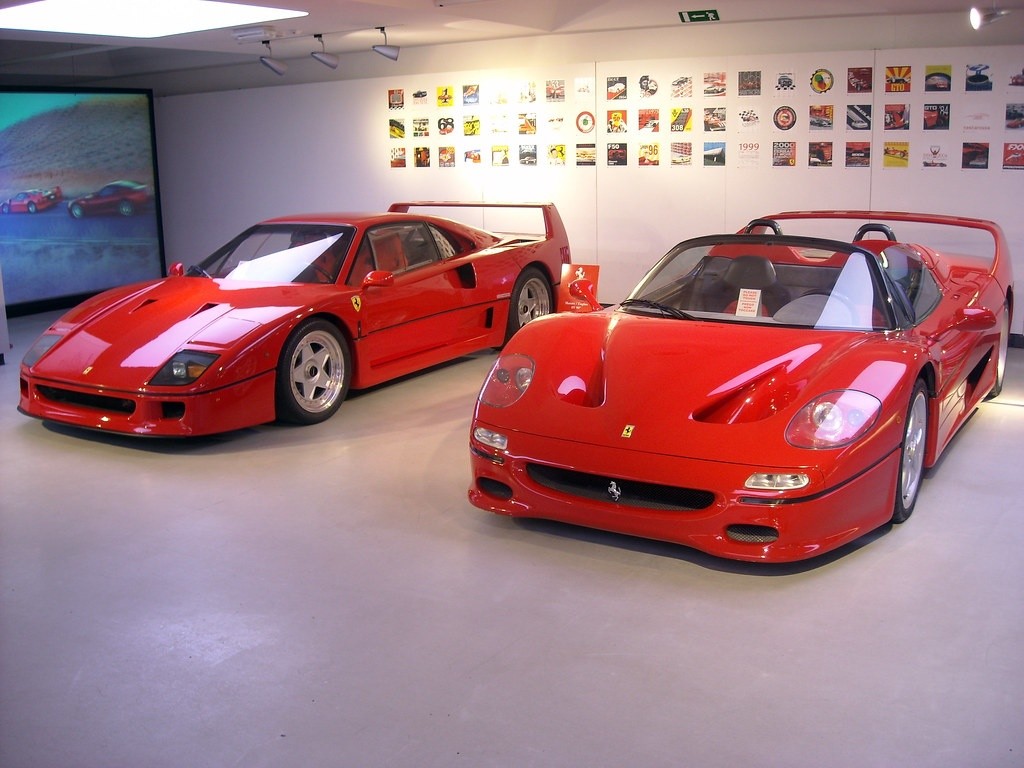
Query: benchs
682,241,939,324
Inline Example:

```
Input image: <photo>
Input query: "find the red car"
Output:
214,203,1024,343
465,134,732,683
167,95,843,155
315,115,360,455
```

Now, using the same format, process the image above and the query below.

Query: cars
520,156,537,165
463,93,478,103
412,90,427,98
672,76,691,87
886,75,910,84
704,77,727,94
1006,118,1024,129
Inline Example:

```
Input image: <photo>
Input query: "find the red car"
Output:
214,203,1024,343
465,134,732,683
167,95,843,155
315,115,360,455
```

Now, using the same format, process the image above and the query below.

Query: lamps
371,27,401,61
970,0,1016,30
259,41,289,75
310,34,339,70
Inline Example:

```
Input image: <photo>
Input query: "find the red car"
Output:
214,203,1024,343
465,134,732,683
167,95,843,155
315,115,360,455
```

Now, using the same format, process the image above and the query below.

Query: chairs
290,232,339,284
348,230,409,285
699,255,791,317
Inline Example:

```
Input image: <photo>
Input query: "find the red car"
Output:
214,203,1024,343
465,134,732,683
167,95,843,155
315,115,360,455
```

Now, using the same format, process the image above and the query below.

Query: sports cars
68,179,155,220
17,201,601,442
467,209,1015,565
0,186,66,218
